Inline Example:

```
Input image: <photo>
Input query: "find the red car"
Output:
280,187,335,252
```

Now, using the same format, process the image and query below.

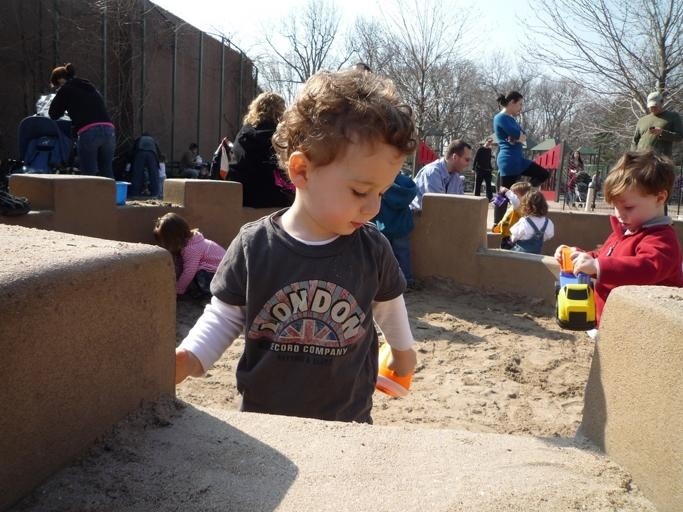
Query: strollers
564,168,596,208
6,111,78,190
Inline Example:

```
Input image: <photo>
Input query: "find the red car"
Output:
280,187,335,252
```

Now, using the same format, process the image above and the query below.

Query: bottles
199,162,208,176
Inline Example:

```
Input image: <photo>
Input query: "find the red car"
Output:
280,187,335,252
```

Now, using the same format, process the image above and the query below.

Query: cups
373,338,413,399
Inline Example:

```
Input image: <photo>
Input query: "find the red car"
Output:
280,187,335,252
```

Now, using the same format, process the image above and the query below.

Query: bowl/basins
115,180,130,205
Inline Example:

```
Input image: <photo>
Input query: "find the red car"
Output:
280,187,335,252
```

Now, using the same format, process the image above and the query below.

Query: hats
485,137,493,142
647,92,662,107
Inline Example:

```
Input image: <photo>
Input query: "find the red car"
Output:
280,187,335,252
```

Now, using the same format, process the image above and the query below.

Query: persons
591,170,603,208
369,169,418,289
46,62,115,177
409,139,472,210
551,148,682,329
472,90,554,253
170,72,418,423
178,142,200,178
152,210,226,301
629,92,682,217
156,154,166,198
565,150,583,206
209,92,295,208
126,132,161,197
